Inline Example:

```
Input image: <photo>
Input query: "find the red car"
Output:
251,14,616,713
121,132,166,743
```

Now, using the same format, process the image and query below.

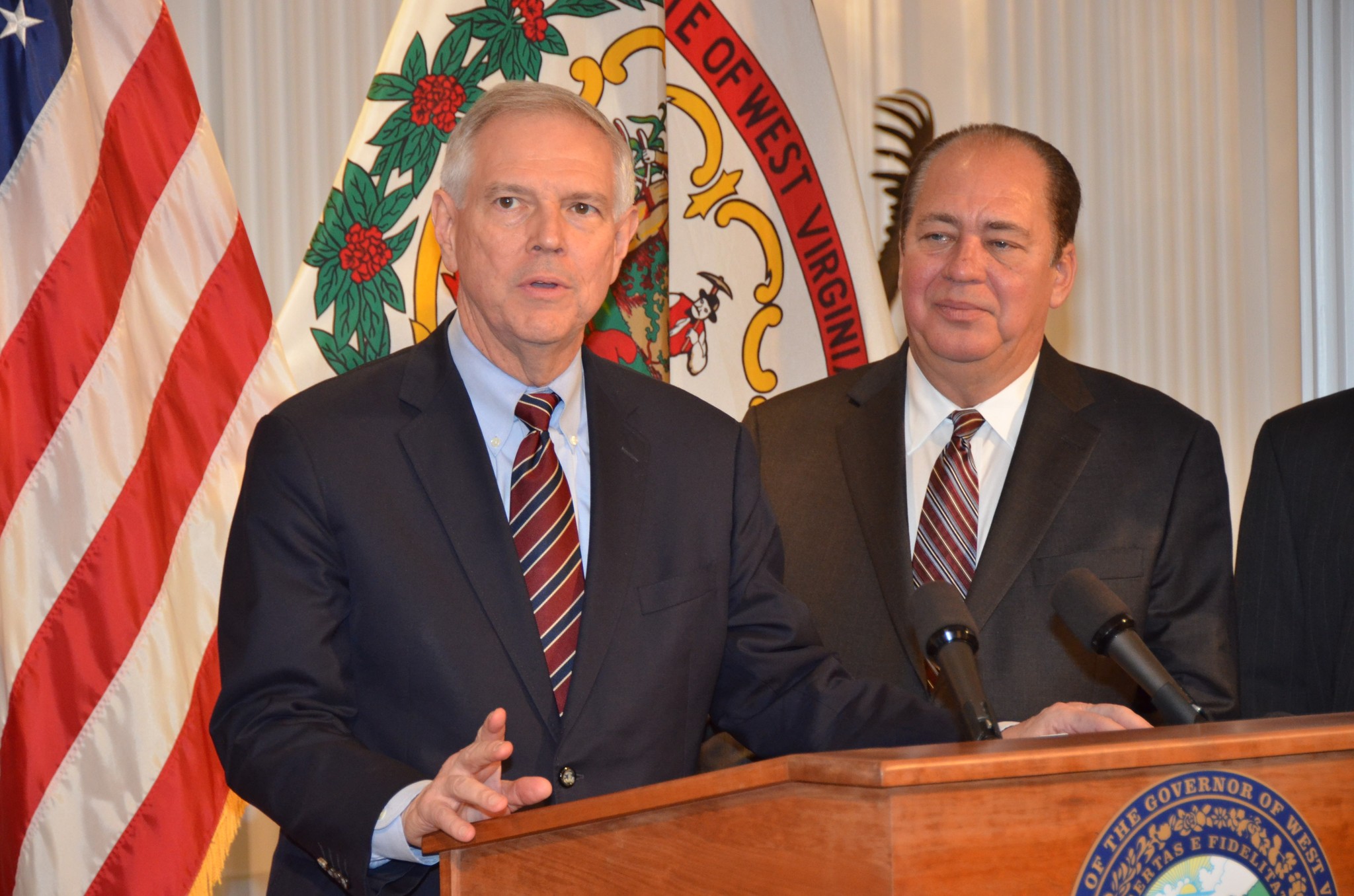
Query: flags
663,0,902,450
264,0,679,403
0,0,324,896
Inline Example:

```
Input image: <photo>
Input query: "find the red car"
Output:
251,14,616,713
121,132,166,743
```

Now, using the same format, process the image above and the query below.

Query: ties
912,409,985,694
506,386,587,721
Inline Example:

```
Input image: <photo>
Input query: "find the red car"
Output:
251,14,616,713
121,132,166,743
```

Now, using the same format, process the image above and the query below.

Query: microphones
908,583,1002,741
1052,567,1215,724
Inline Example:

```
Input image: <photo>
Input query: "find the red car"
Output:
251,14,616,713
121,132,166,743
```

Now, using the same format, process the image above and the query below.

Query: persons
201,79,1163,896
1232,384,1353,734
696,119,1240,770
